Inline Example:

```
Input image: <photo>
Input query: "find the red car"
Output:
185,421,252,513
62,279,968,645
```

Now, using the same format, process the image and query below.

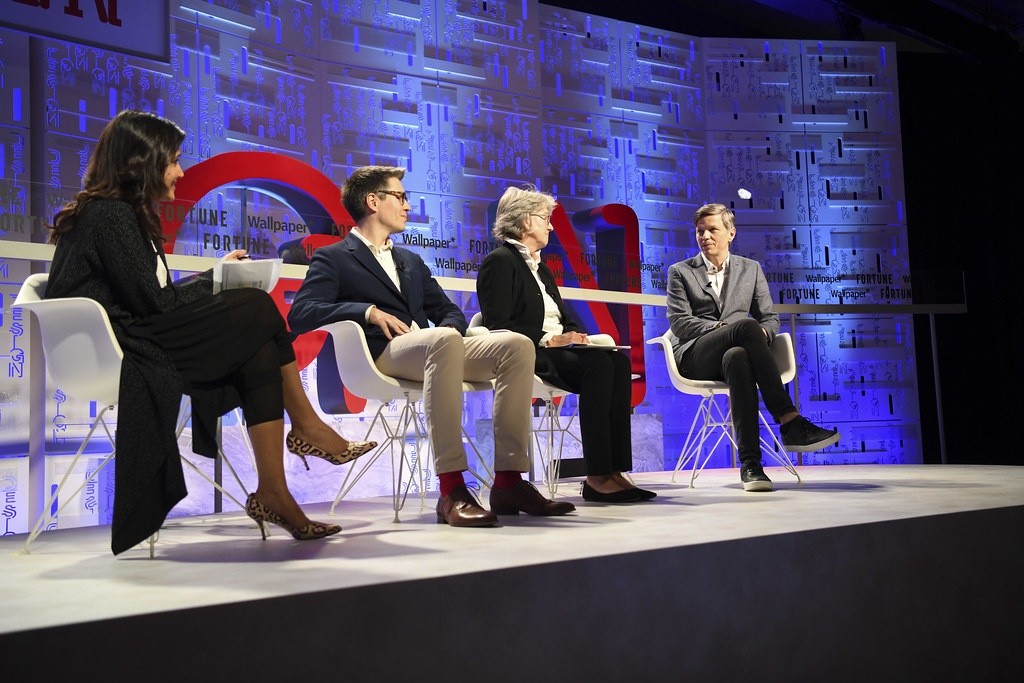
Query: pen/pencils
236,255,250,260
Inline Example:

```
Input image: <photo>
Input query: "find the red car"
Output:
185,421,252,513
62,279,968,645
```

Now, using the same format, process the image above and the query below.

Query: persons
286,165,577,531
476,183,658,505
43,108,378,559
666,203,841,493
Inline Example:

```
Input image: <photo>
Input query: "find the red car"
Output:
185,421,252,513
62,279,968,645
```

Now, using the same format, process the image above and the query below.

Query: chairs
645,323,806,488
462,311,642,499
308,316,549,528
9,271,275,560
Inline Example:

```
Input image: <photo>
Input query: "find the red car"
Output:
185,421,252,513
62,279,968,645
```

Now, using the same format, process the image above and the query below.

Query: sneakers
740,466,773,491
780,415,841,452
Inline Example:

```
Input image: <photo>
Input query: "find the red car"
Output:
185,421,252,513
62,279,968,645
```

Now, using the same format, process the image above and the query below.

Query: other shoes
582,481,656,502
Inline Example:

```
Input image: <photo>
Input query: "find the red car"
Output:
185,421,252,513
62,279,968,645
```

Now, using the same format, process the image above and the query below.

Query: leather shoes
436,483,498,527
490,480,576,516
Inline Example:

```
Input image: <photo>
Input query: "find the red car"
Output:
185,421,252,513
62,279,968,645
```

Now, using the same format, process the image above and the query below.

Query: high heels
247,494,342,541
286,431,378,470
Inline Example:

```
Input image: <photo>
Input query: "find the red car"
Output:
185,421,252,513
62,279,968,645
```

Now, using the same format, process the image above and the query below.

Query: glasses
530,213,550,224
372,190,408,204
